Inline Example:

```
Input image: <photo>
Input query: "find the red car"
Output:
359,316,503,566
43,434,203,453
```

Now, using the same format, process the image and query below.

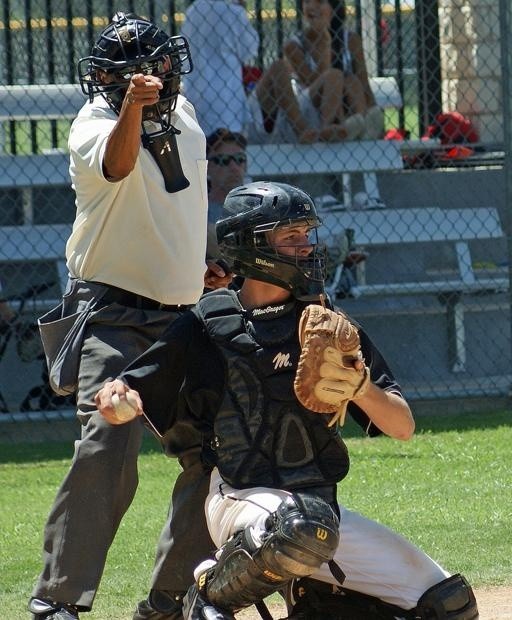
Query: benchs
1,77,510,372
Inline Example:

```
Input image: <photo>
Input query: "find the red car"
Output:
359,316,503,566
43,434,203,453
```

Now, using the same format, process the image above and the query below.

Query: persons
92,179,480,620
282,0,387,142
29,16,240,619
202,129,248,292
178,0,262,142
237,55,348,144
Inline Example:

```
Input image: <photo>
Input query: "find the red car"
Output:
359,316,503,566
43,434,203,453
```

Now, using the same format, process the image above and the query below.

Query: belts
100,286,196,313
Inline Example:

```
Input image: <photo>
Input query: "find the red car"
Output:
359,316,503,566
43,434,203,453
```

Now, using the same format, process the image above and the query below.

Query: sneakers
287,578,333,618
343,114,365,141
181,582,237,620
357,105,385,141
315,195,345,212
351,192,386,210
26,599,80,619
131,596,185,619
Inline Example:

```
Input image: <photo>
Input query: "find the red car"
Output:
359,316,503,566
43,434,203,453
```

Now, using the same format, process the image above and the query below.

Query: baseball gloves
294,304,371,415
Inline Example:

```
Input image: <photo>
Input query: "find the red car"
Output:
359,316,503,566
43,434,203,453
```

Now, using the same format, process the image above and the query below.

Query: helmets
215,180,328,304
77,11,194,136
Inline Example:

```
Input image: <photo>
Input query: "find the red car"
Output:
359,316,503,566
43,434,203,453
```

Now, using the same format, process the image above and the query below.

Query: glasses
208,153,247,167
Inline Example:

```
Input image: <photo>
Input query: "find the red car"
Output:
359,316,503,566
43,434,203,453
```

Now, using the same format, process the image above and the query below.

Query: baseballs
110,392,142,423
